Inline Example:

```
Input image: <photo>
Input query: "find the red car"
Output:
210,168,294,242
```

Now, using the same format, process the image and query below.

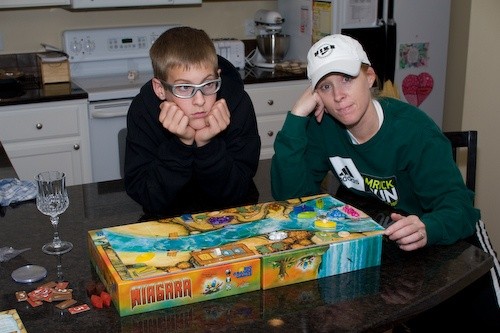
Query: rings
418,231,424,240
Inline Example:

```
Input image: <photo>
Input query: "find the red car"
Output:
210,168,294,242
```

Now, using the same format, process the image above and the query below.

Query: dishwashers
60,25,186,182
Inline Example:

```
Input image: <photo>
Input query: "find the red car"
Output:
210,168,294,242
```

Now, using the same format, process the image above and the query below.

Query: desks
0,159,495,333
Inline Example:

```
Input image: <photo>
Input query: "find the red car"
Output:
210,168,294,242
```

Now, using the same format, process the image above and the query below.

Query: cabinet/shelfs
0,75,93,185
242,67,309,160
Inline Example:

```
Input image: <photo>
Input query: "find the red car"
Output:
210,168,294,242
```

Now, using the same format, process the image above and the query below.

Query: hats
306,34,371,94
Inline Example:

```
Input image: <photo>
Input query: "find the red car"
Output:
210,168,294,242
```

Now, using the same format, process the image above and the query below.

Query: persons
270,34,477,251
123,25,261,209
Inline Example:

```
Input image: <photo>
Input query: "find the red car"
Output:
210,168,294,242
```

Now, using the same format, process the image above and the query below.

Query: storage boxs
87,193,386,317
36,52,72,84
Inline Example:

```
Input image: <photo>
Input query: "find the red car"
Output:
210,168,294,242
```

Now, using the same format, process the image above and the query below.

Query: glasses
160,75,221,98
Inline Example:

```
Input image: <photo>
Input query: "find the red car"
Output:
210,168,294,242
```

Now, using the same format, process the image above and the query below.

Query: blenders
250,9,291,68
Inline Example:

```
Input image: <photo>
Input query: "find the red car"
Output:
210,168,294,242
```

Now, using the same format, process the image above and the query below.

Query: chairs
443,130,478,192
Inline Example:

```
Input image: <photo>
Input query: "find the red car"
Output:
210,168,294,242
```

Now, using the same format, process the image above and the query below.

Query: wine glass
35,171,73,255
